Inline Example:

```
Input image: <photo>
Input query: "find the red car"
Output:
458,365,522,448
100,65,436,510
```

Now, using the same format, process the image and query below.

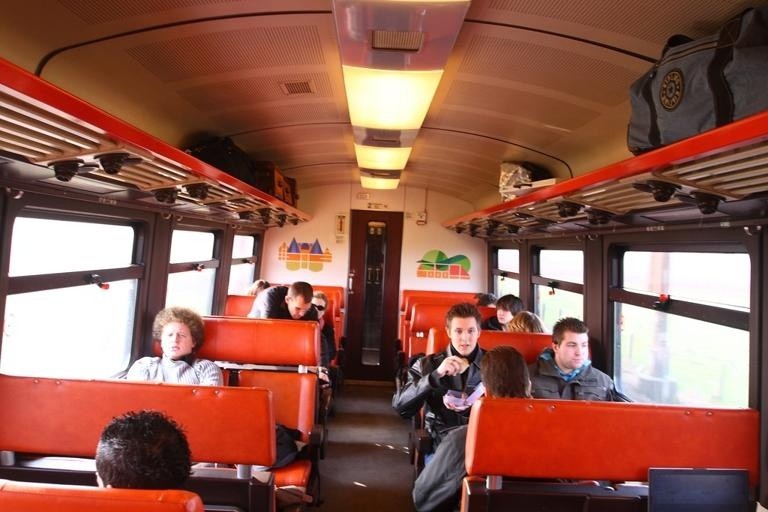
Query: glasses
312,304,326,311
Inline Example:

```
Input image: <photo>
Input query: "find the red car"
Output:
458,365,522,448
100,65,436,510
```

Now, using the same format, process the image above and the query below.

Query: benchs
224,282,345,409
460,397,760,510
409,326,589,471
1,372,275,511
397,288,589,359
150,312,323,503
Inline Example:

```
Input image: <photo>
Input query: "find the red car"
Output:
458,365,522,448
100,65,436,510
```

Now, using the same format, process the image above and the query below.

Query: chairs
1,476,206,511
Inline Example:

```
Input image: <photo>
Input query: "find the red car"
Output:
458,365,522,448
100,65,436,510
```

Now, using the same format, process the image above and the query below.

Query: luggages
255,166,294,206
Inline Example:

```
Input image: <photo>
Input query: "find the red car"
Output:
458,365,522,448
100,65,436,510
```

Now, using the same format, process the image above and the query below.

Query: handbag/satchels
252,424,301,471
628,7,764,157
184,135,257,187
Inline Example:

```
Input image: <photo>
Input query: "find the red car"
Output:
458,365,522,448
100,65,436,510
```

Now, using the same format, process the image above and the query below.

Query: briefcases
499,178,563,195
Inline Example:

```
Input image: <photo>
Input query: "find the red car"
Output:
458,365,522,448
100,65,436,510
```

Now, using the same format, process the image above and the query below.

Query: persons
392,303,490,448
412,347,533,512
246,280,270,296
477,294,497,308
128,306,224,387
94,409,193,488
482,294,523,331
528,317,622,489
247,282,318,321
507,312,545,334
310,291,336,360
